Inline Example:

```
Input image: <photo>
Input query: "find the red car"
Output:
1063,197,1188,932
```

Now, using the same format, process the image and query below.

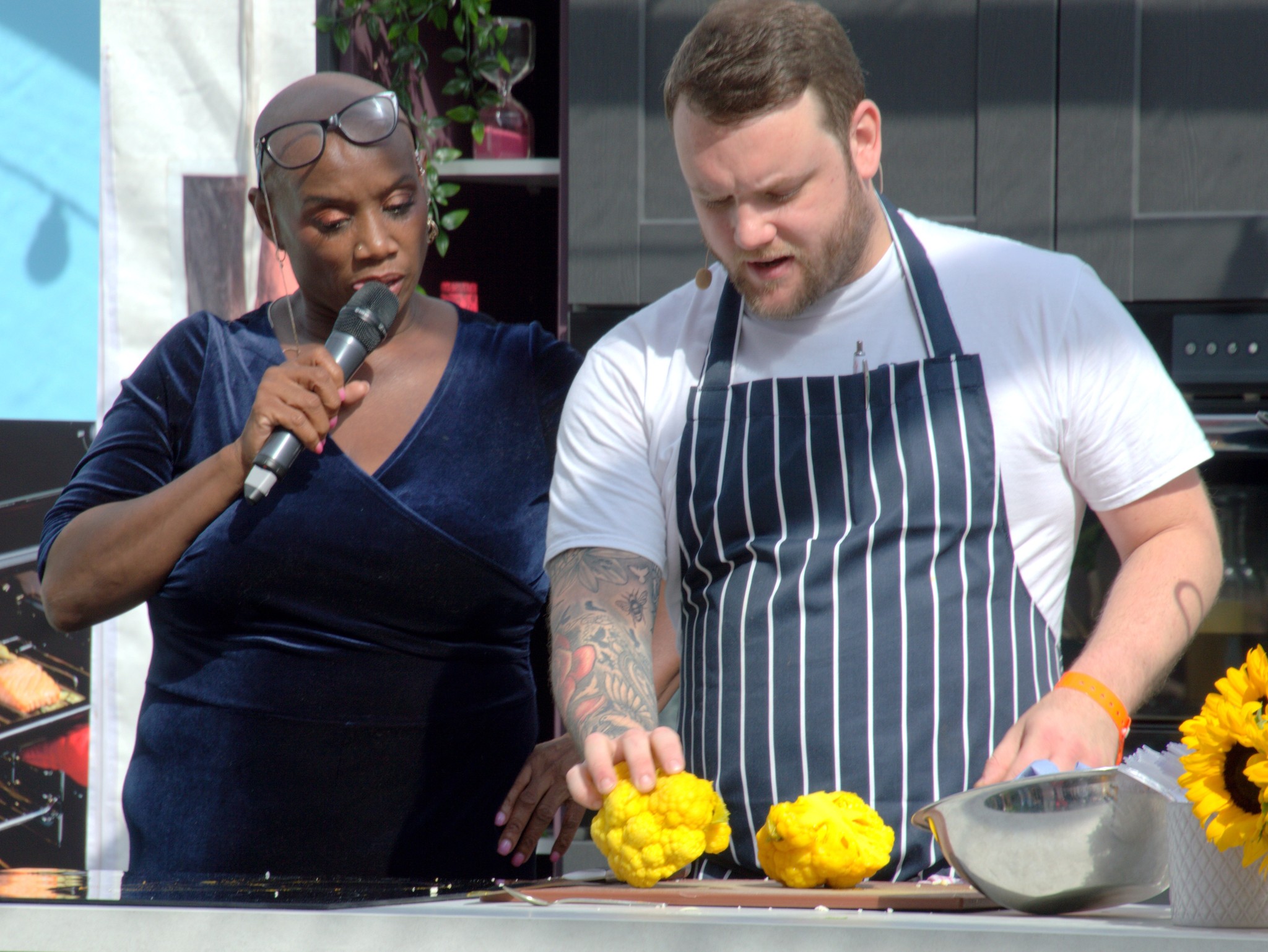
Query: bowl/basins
909,767,1178,913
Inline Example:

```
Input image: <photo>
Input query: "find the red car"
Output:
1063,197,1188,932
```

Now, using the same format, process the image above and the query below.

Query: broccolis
755,790,896,889
588,763,731,889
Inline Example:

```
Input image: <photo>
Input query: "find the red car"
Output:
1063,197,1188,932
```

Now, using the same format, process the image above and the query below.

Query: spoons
502,885,665,907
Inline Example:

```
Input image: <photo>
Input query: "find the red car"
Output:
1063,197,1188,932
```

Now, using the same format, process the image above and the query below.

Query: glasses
256,91,418,191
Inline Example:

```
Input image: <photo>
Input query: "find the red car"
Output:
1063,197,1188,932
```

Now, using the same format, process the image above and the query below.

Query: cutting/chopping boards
478,873,1001,912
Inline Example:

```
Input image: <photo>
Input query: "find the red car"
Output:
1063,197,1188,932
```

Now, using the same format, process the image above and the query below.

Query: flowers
1171,640,1268,882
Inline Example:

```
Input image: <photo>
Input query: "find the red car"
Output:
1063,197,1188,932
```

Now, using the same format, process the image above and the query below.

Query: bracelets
1055,671,1133,739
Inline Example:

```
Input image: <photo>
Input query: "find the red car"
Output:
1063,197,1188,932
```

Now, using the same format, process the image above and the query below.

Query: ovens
1057,300,1268,757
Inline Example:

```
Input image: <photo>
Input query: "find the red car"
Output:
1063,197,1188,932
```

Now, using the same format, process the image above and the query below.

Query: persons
34,69,684,884
541,1,1225,881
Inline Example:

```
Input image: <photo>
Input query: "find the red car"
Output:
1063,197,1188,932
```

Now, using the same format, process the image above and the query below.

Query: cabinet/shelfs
564,0,1268,311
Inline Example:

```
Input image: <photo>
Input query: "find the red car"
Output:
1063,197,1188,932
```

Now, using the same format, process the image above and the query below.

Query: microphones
245,281,400,507
